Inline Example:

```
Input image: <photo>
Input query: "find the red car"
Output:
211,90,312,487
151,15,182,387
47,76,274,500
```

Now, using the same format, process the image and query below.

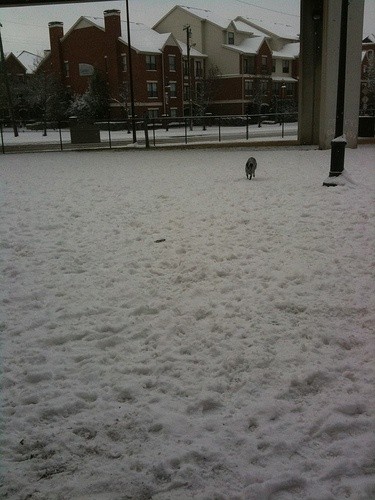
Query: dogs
245,157,258,181
